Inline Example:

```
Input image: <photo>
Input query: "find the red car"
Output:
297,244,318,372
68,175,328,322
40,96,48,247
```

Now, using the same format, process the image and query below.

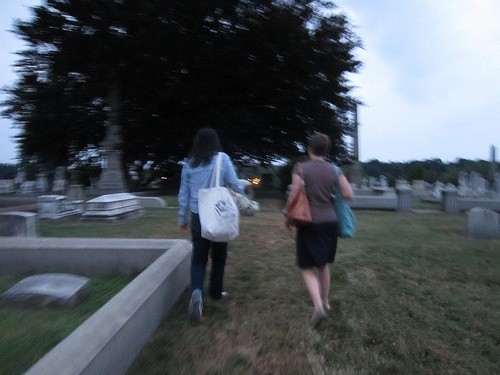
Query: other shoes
309,308,328,329
324,304,332,314
221,292,228,299
189,288,203,319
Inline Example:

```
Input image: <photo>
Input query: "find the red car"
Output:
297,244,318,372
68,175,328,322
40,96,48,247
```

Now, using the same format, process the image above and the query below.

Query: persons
174,125,246,324
282,129,356,328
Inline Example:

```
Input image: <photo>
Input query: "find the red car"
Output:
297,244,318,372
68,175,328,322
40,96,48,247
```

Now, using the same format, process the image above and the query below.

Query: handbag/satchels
334,165,356,237
287,162,312,223
198,152,240,242
